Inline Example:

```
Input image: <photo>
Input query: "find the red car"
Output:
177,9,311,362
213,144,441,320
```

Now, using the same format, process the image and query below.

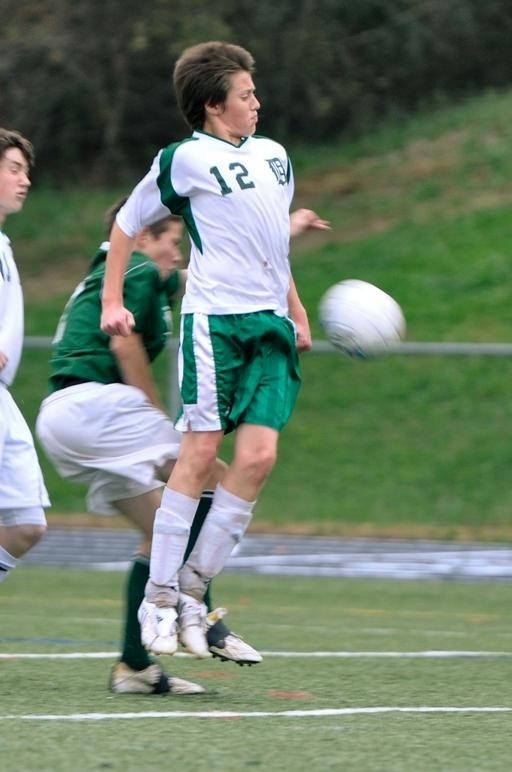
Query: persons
34,192,333,696
99,40,314,657
0,127,54,576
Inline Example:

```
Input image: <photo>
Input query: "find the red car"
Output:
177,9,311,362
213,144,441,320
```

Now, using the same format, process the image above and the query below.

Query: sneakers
110,663,204,697
176,594,209,655
205,609,262,666
137,599,176,656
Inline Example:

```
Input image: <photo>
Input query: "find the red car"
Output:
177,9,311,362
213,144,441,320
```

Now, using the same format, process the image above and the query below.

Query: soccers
317,279,406,362
